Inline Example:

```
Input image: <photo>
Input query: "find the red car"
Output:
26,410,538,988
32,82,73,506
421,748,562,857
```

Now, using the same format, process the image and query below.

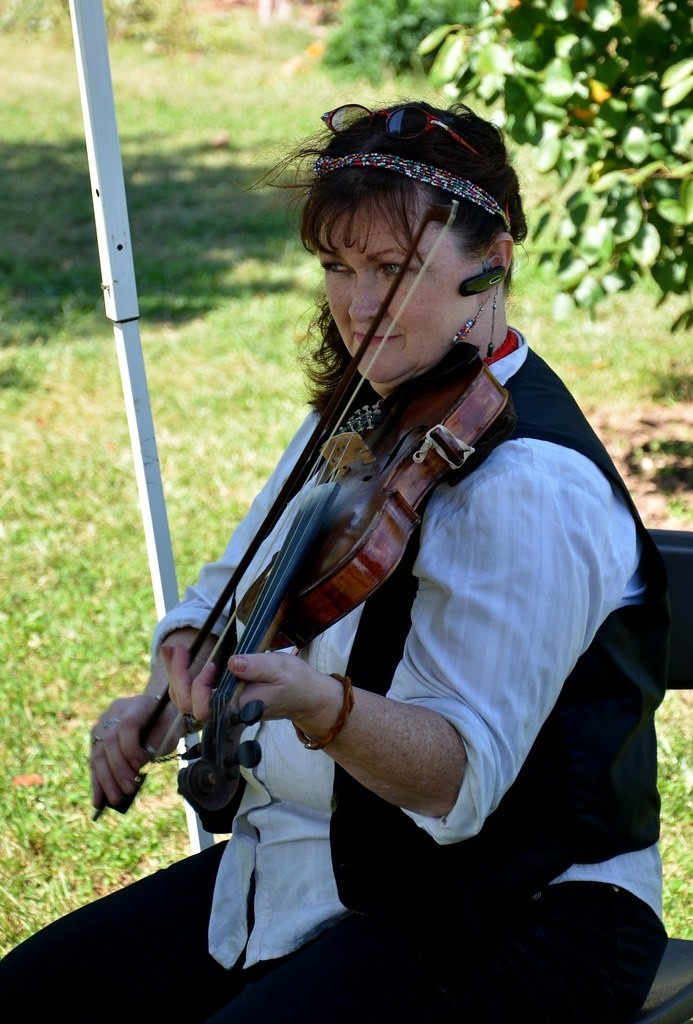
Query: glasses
320,103,481,158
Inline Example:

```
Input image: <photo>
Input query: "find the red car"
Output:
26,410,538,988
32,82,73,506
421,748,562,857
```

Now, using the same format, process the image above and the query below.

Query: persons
0,98,671,1024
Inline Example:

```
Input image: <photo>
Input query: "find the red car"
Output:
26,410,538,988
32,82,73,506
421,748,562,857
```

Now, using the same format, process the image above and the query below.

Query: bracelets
293,670,354,749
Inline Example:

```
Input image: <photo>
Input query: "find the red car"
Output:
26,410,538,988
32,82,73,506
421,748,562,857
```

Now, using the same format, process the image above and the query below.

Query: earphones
461,266,506,299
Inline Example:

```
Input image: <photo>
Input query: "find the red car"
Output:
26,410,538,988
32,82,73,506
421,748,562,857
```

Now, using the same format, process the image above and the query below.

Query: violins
173,341,510,835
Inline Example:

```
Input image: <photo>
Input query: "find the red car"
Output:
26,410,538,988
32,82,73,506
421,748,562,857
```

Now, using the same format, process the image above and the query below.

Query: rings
91,735,103,744
103,718,119,728
146,745,157,755
